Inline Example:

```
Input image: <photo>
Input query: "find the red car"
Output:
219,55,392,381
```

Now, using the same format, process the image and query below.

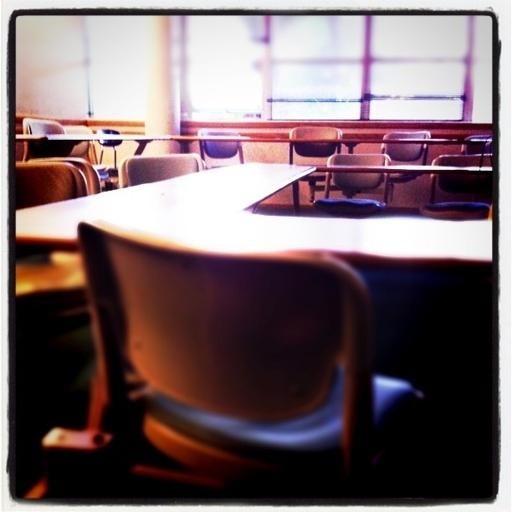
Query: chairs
14,118,494,499
